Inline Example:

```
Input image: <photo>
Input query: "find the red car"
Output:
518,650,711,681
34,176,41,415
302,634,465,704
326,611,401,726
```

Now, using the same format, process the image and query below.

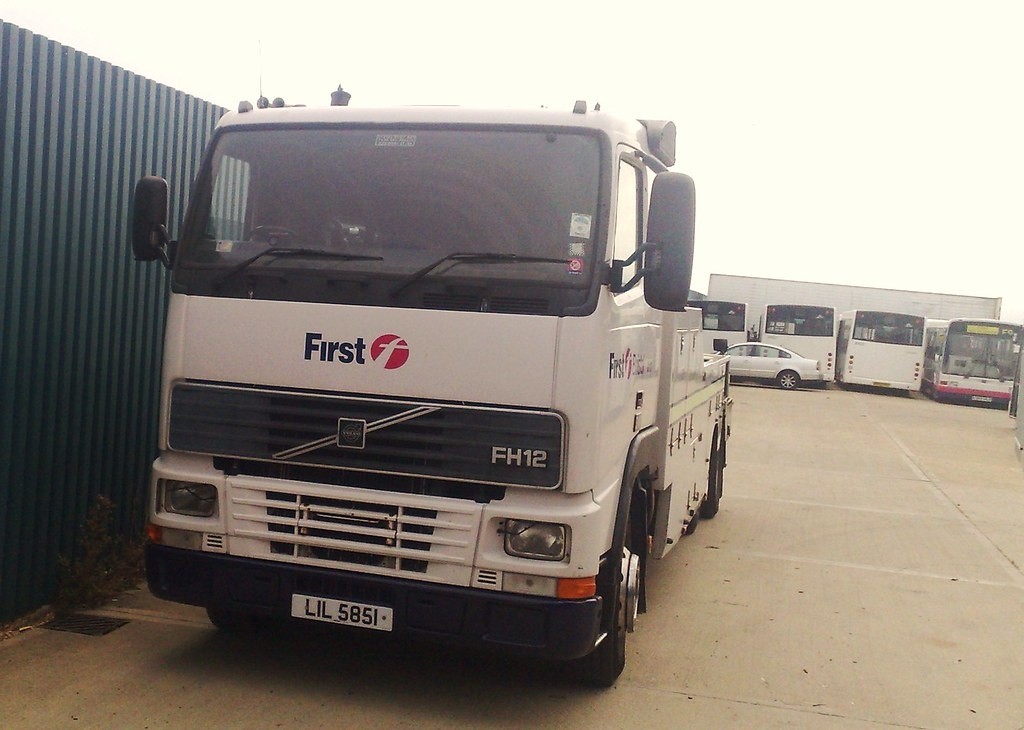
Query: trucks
124,81,734,701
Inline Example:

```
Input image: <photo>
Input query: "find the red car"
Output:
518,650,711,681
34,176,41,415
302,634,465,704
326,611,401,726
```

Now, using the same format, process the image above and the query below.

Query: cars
716,342,821,391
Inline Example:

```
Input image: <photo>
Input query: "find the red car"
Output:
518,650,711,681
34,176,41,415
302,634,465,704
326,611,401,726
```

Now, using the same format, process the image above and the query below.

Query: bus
923,317,1024,409
754,303,837,388
835,309,928,398
685,299,751,365
